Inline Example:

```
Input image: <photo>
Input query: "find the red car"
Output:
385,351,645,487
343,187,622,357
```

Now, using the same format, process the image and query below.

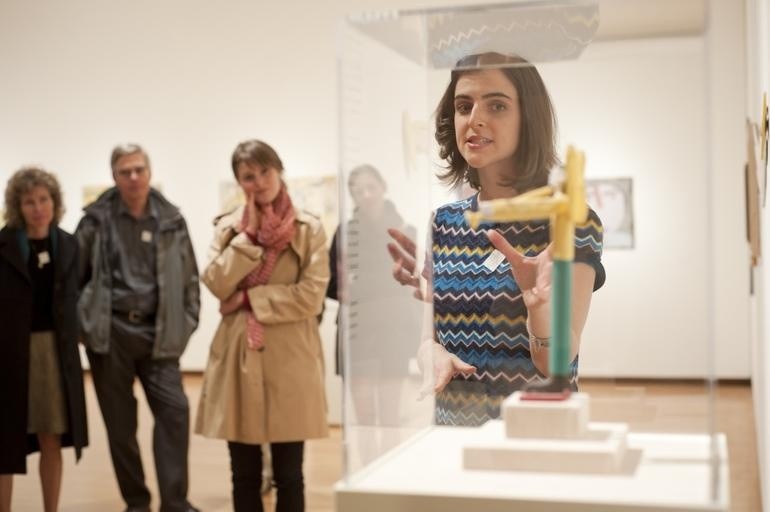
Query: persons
417,51,605,426
194,138,332,511
325,164,423,426
0,168,89,512
74,144,201,511
387,228,433,304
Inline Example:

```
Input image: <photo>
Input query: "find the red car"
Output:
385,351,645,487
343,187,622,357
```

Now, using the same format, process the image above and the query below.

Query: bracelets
523,315,552,349
